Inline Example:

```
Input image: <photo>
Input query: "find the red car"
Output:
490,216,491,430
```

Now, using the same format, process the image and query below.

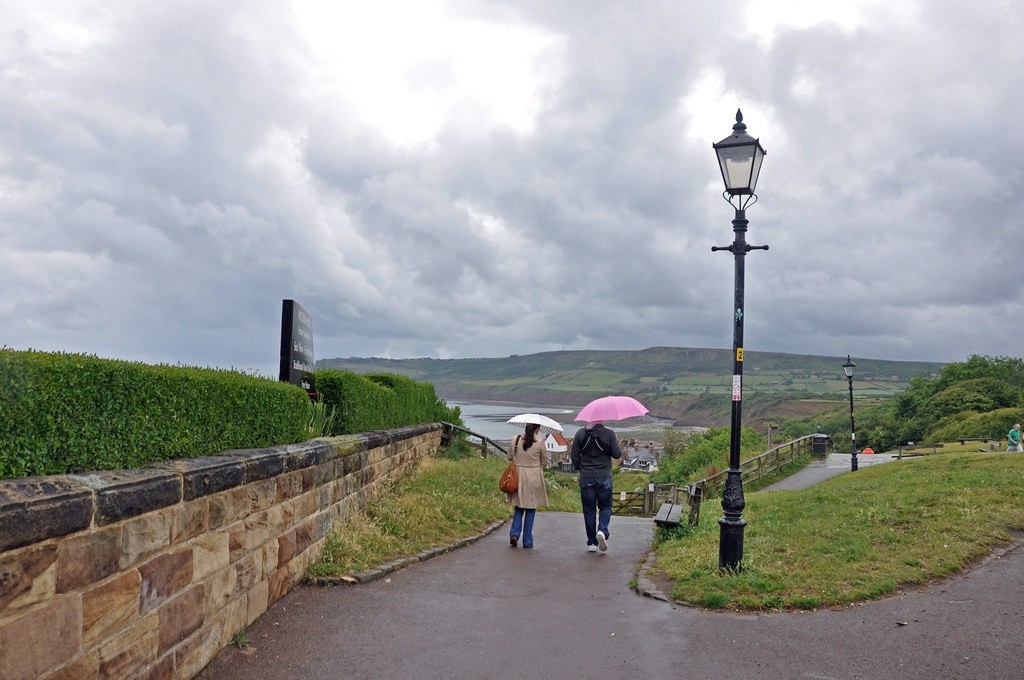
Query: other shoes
509,536,518,547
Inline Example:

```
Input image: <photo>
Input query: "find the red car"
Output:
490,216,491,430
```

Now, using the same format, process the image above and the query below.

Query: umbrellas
574,393,650,431
505,413,563,435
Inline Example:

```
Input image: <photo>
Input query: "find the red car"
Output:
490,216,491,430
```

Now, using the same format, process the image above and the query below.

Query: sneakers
595,530,610,551
587,543,598,552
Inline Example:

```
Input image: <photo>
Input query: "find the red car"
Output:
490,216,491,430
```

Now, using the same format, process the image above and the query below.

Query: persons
1006,424,1023,452
862,445,875,454
507,423,550,548
571,420,622,551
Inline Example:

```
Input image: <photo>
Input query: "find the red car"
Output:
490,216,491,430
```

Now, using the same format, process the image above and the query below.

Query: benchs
955,437,993,445
890,444,944,460
654,482,704,537
980,440,1003,454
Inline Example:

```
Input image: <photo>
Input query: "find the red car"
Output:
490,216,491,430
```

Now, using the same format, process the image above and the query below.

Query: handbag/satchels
499,434,522,495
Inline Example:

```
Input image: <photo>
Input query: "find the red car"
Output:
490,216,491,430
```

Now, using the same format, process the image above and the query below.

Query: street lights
711,106,771,579
843,355,859,472
